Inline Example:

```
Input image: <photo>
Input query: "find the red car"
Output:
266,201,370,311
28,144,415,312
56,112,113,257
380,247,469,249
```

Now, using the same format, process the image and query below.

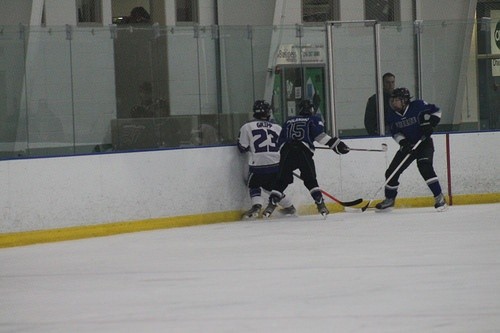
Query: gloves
419,118,433,137
328,137,350,154
399,139,416,156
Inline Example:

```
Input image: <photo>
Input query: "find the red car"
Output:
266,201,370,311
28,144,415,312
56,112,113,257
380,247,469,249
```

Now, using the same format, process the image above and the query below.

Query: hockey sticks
312,143,388,153
344,129,434,213
292,172,363,207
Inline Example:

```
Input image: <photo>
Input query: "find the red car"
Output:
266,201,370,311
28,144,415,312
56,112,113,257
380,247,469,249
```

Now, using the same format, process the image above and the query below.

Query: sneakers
318,206,331,216
434,194,447,209
260,203,275,217
376,199,395,209
278,205,297,216
240,203,262,219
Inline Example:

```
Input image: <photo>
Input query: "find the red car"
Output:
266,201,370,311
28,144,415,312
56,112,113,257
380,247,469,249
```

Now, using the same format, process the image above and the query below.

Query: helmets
253,100,272,119
296,99,315,116
389,87,410,100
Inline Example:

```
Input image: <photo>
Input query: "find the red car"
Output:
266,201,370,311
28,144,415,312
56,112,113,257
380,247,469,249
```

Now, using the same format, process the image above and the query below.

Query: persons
376,87,449,212
365,73,396,137
237,99,297,220
114,7,164,119
261,99,351,220
